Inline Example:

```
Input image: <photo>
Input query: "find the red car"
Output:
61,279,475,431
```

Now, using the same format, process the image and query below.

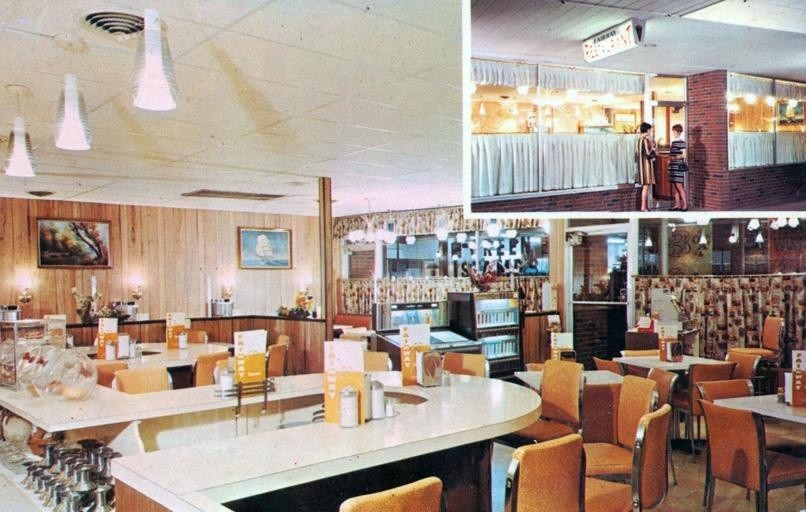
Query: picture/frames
34,215,114,270
775,99,806,127
237,224,294,271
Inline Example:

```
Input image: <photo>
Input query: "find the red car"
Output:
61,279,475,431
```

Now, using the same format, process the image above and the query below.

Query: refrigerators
447,291,524,385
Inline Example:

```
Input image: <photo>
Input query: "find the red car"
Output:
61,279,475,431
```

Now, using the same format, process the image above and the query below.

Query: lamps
52,34,95,152
1,83,40,180
129,4,178,113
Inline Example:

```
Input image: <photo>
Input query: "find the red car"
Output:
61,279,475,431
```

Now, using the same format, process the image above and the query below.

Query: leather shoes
679,204,690,211
669,206,680,211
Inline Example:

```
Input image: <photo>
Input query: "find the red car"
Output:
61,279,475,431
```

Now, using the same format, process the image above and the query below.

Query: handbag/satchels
678,162,689,172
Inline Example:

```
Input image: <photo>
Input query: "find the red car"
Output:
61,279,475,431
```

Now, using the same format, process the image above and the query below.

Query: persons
657,125,690,211
634,122,656,211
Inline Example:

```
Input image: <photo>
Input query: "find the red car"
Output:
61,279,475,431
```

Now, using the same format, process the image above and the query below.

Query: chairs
0,313,806,510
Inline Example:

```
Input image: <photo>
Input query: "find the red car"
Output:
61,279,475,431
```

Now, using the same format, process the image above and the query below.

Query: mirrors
343,212,550,285
636,217,805,281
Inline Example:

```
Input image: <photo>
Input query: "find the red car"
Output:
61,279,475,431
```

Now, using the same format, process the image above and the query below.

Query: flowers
71,285,124,323
288,286,315,314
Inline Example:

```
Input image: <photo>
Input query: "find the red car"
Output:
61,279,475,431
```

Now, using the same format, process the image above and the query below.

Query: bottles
214,360,234,399
647,312,659,333
178,331,188,348
106,332,143,360
111,301,138,322
266,378,271,391
442,370,450,385
66,334,74,347
212,299,232,317
277,296,315,319
0,415,122,512
0,304,21,321
339,374,396,428
779,387,785,402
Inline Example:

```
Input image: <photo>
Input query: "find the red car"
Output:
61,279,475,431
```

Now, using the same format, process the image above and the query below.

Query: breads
26,380,86,401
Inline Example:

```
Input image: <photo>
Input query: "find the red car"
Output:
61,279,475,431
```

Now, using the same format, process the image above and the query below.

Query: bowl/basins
1,338,97,401
